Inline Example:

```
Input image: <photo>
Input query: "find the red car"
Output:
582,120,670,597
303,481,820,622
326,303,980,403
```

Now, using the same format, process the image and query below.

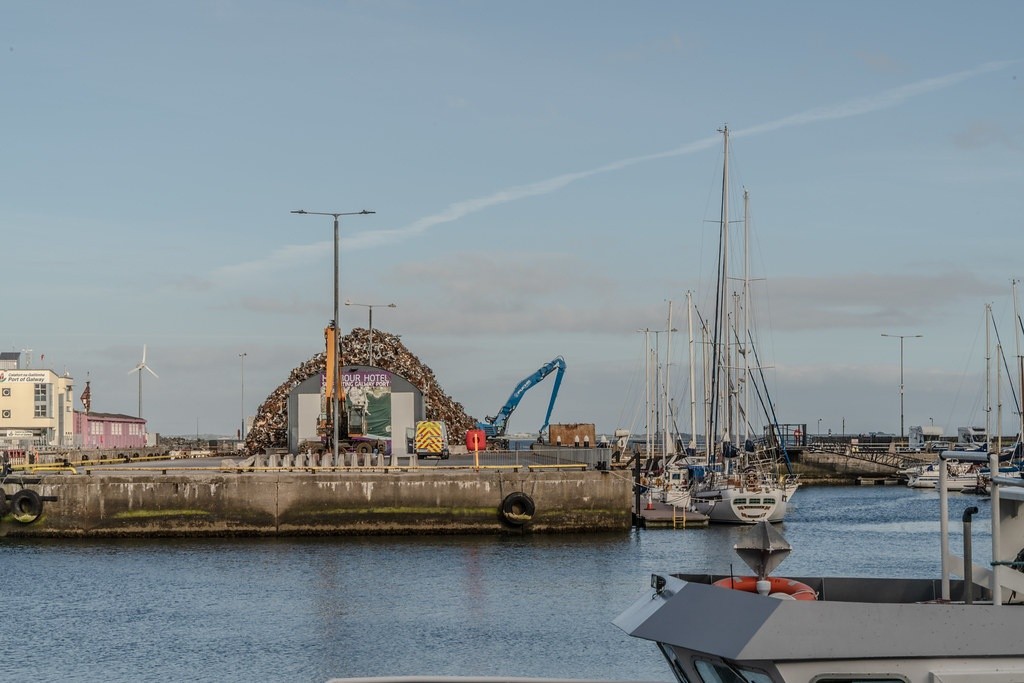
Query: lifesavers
10,490,43,523
794,429,801,439
711,576,818,601
502,492,535,524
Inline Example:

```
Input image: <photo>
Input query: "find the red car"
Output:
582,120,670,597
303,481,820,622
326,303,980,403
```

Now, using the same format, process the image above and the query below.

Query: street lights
344,301,397,366
881,333,924,438
239,353,248,439
291,209,379,466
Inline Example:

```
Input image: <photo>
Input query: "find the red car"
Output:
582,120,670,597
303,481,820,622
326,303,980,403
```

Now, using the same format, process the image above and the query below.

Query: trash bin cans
3,463,12,474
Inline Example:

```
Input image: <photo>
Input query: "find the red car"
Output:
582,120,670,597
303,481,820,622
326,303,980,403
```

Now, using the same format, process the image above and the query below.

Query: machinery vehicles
475,355,567,450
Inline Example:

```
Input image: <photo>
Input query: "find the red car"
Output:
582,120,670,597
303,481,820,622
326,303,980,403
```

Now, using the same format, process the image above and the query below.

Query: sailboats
606,122,1024,526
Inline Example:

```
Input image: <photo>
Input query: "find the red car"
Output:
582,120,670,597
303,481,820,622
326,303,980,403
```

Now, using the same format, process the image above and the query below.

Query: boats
609,449,1024,683
